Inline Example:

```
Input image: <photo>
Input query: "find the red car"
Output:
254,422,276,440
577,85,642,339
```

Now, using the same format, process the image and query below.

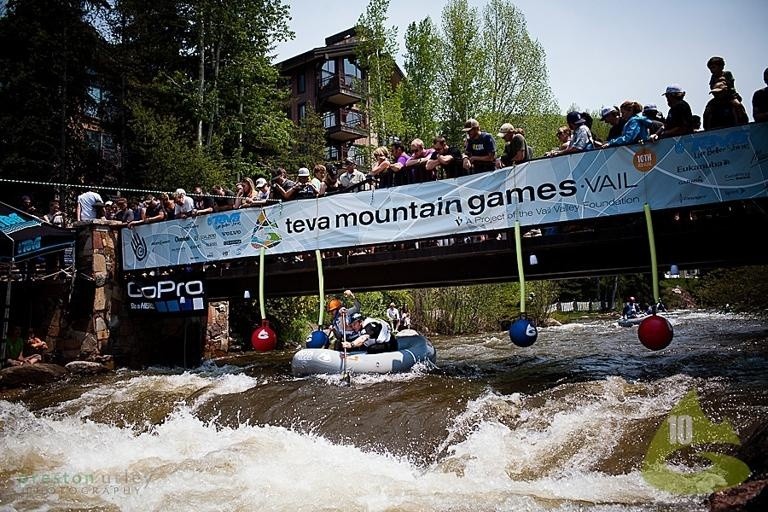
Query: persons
623,294,644,319
325,287,411,354
0,55,767,231
24,324,49,363
6,324,27,366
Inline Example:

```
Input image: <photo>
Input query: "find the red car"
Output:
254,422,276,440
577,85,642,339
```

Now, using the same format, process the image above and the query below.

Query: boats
618,309,662,327
292,328,437,379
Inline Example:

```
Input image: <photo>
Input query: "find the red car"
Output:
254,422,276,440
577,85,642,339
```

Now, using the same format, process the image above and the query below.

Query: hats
327,299,340,311
343,119,515,165
348,312,362,325
21,167,310,207
567,81,727,127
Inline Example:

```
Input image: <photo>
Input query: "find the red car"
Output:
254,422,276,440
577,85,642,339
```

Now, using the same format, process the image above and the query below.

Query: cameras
31,206,36,211
60,211,68,217
272,181,277,189
299,184,306,188
